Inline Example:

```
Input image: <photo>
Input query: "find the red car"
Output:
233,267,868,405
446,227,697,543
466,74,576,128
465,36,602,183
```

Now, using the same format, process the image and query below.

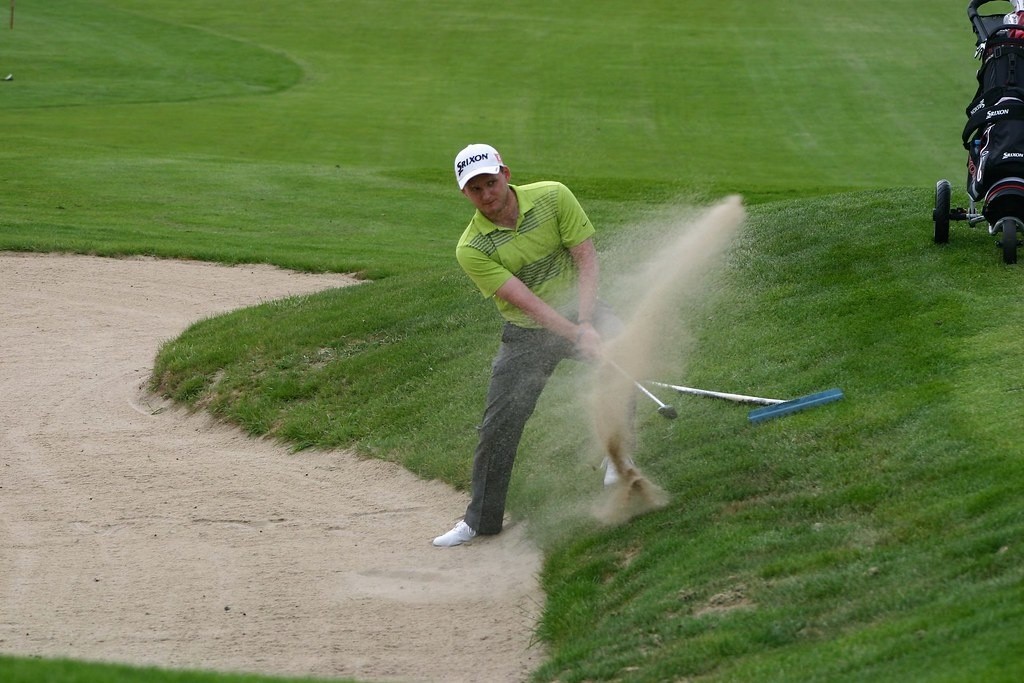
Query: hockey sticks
599,350,680,421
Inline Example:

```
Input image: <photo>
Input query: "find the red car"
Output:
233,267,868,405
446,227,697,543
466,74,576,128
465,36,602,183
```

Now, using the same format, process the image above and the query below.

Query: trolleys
932,1,1023,265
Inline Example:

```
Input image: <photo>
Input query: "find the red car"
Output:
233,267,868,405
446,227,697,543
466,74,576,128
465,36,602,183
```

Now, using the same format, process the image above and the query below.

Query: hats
454,143,504,190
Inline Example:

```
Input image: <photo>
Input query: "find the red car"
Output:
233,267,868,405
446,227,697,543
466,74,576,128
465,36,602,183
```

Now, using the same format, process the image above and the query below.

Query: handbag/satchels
962,85,1024,202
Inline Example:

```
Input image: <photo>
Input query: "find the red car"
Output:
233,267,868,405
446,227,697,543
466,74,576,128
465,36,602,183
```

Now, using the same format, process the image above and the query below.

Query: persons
433,144,632,548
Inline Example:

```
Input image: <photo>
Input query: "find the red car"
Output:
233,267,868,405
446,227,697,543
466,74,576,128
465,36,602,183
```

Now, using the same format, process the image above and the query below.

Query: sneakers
599,453,634,486
433,519,480,547
